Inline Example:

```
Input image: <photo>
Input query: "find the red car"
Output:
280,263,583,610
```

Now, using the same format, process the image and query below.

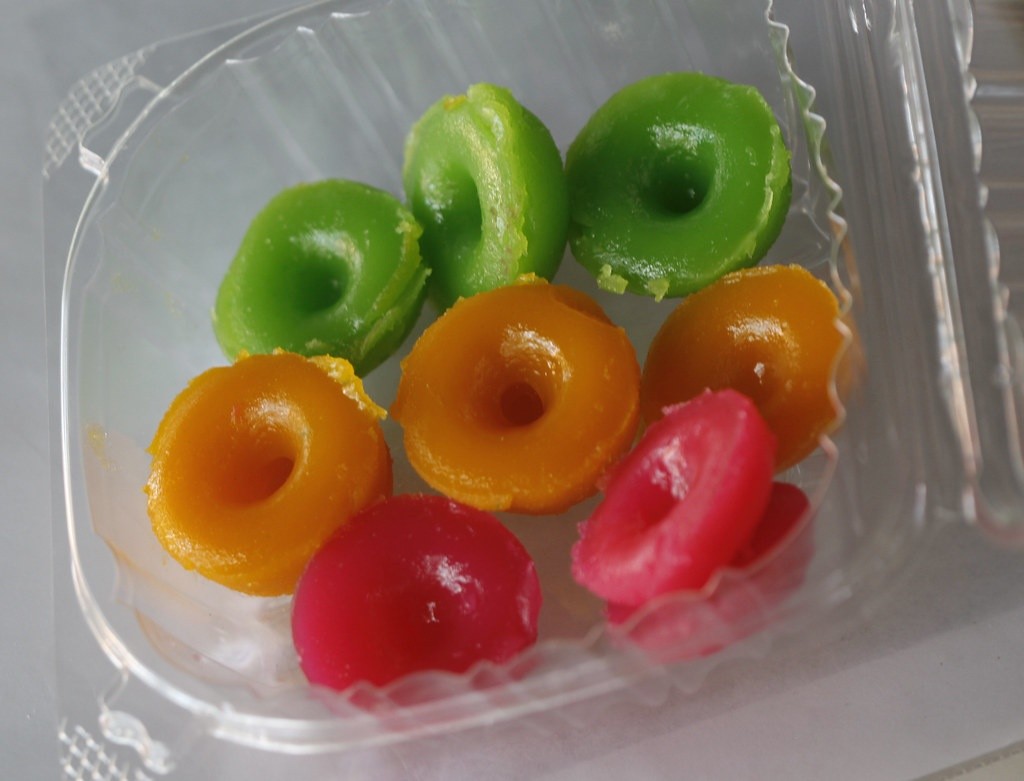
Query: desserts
144,71,859,711
